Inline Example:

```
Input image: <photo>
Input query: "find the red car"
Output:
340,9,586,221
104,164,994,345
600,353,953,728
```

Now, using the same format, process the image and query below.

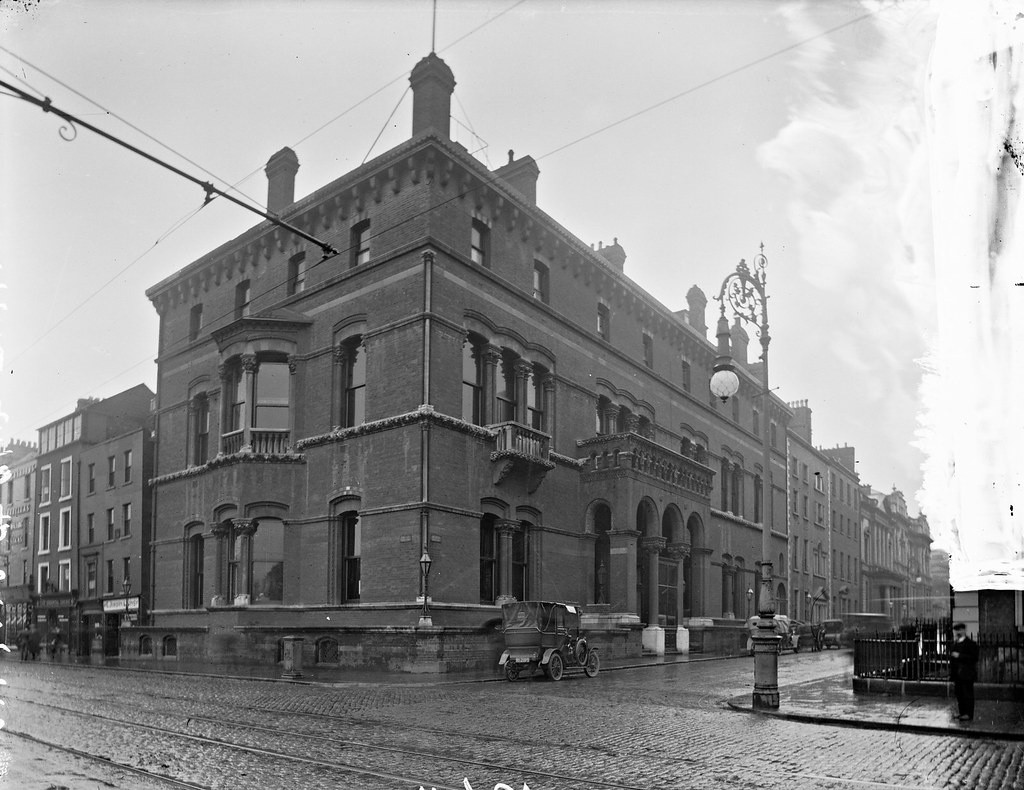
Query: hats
953,624,965,630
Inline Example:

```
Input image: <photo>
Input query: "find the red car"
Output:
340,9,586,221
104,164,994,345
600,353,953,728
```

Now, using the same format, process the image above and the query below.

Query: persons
16,624,39,661
950,624,979,722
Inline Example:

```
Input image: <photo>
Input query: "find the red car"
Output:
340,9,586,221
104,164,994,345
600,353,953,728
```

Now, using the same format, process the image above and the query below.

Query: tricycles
498,601,602,683
823,619,847,651
745,616,802,656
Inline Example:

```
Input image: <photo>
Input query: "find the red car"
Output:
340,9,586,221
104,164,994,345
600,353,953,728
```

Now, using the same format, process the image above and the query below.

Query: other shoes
959,714,973,723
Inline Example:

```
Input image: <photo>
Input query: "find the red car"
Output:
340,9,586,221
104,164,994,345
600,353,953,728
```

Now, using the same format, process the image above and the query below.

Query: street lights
710,241,782,712
